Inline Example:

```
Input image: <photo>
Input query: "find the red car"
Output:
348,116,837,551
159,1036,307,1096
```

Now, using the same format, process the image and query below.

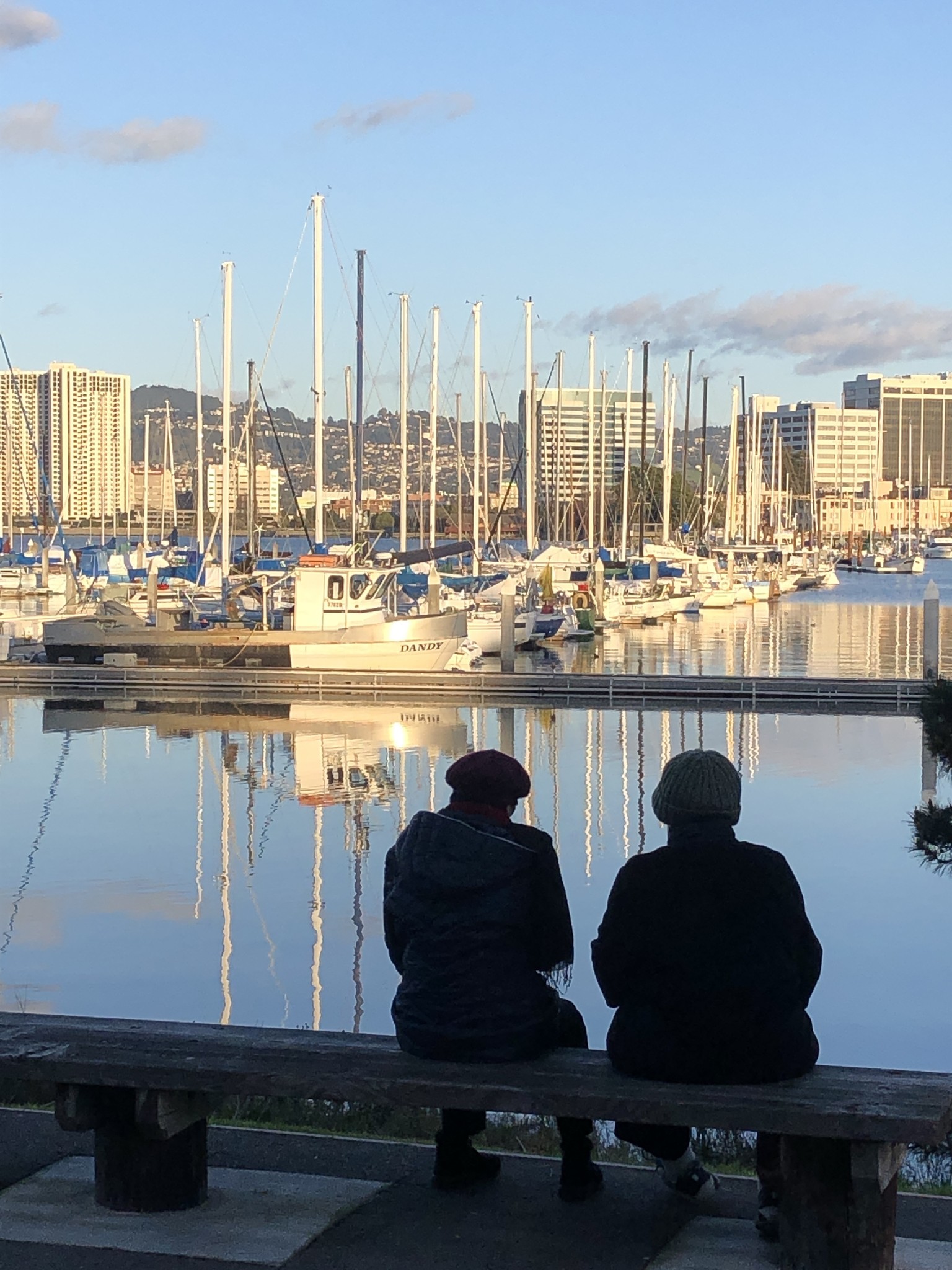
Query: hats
445,748,531,799
652,748,742,826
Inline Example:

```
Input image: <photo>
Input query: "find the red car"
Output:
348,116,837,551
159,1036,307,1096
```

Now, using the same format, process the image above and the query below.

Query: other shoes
557,1162,604,1202
433,1148,500,1184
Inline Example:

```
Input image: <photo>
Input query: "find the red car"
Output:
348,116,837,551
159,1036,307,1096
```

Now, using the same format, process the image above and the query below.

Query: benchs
25,1028,952,1270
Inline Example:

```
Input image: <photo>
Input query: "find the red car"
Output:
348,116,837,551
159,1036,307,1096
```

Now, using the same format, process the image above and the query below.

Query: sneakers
754,1190,784,1238
662,1160,720,1205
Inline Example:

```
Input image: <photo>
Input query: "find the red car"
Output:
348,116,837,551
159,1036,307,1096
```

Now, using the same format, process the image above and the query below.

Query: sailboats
0,193,952,673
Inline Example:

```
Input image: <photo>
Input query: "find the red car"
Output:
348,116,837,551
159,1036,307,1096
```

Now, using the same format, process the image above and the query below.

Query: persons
379,747,608,1203
590,748,826,1239
